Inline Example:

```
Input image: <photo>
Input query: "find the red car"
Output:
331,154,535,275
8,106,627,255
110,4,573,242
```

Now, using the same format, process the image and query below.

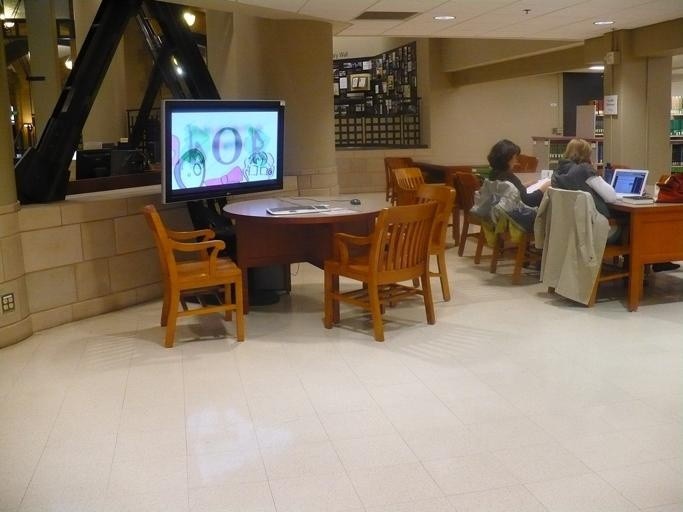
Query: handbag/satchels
653,175,683,203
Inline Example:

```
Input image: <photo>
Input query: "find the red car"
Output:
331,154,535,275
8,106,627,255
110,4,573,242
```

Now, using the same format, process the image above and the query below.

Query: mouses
351,199,362,206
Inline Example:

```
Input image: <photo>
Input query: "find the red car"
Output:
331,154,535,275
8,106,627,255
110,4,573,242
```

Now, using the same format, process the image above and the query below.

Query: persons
485,138,551,273
550,136,680,288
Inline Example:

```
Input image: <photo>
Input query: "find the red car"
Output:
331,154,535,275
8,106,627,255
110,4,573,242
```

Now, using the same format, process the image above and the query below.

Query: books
669,96,682,175
548,140,603,177
586,99,603,138
621,194,655,206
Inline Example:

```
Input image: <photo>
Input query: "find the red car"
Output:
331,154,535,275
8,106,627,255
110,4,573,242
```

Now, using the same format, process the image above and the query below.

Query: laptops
610,169,650,198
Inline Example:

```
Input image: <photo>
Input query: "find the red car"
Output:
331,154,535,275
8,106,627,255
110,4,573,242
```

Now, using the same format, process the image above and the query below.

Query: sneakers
653,263,681,271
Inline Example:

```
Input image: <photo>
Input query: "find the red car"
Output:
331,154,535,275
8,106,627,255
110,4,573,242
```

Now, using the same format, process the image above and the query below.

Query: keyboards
266,205,329,215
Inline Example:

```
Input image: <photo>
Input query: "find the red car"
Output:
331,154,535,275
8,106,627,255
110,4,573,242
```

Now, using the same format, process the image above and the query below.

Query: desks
607,192,682,312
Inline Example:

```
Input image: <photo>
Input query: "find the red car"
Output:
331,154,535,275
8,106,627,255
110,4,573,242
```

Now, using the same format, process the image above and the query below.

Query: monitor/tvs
159,99,286,205
